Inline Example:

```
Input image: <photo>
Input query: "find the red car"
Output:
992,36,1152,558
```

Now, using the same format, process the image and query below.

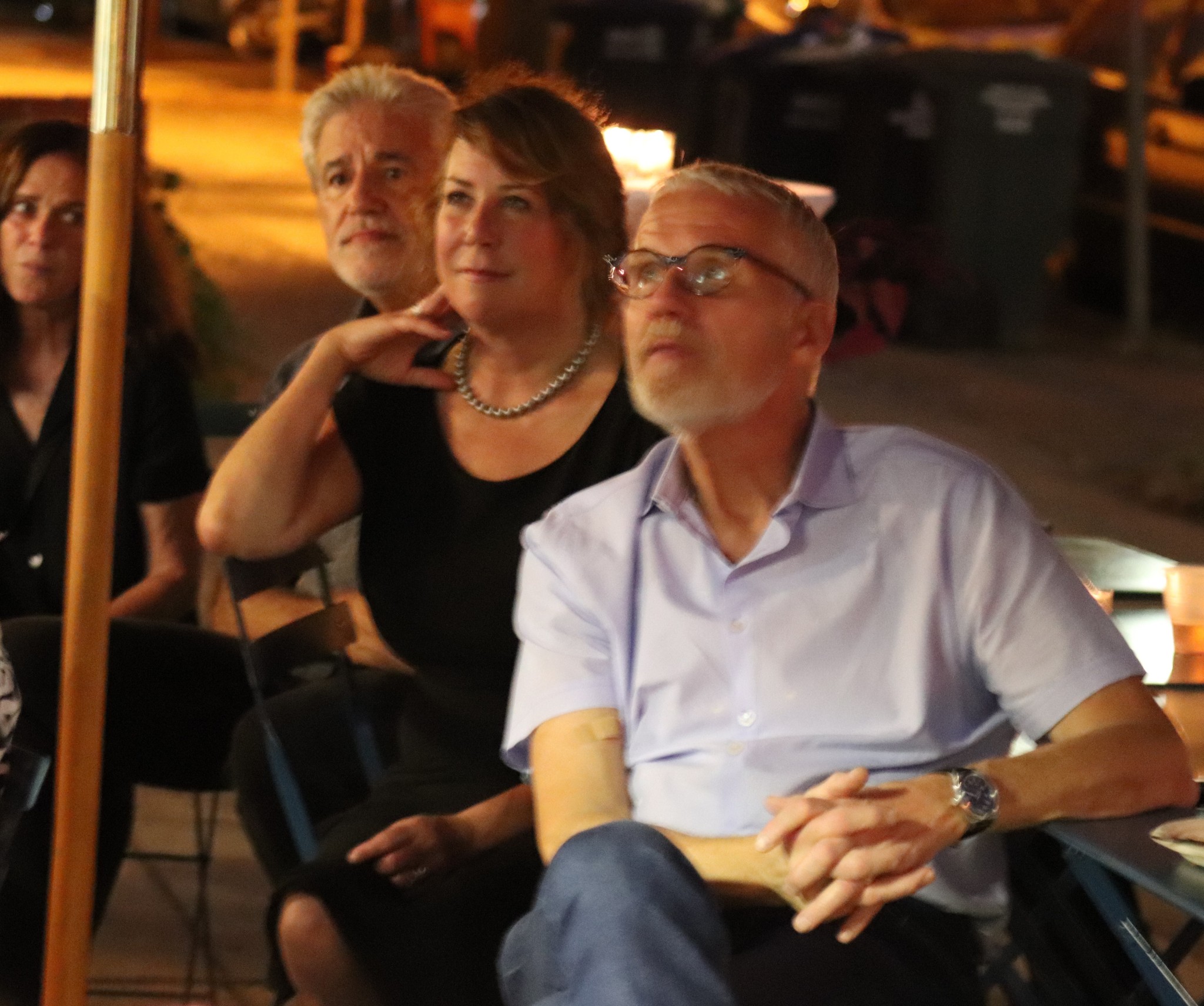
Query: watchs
932,764,1000,842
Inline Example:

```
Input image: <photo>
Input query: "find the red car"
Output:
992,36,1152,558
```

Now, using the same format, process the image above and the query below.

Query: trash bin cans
711,45,1090,354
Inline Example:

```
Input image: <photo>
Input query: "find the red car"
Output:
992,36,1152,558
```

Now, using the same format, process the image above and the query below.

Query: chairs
28,399,1204,1006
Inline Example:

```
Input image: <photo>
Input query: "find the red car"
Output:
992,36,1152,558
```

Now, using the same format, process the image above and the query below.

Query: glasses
604,243,814,303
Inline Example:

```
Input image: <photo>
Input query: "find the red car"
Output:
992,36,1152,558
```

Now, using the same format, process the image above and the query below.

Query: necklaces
453,308,605,419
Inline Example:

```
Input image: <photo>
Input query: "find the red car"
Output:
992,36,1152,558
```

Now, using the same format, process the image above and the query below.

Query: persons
190,75,681,1006
1,62,505,1006
498,161,1197,1005
1,114,215,1006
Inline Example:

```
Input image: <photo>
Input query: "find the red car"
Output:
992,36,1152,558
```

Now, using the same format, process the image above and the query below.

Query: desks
1035,779,1204,1006
697,47,1097,341
